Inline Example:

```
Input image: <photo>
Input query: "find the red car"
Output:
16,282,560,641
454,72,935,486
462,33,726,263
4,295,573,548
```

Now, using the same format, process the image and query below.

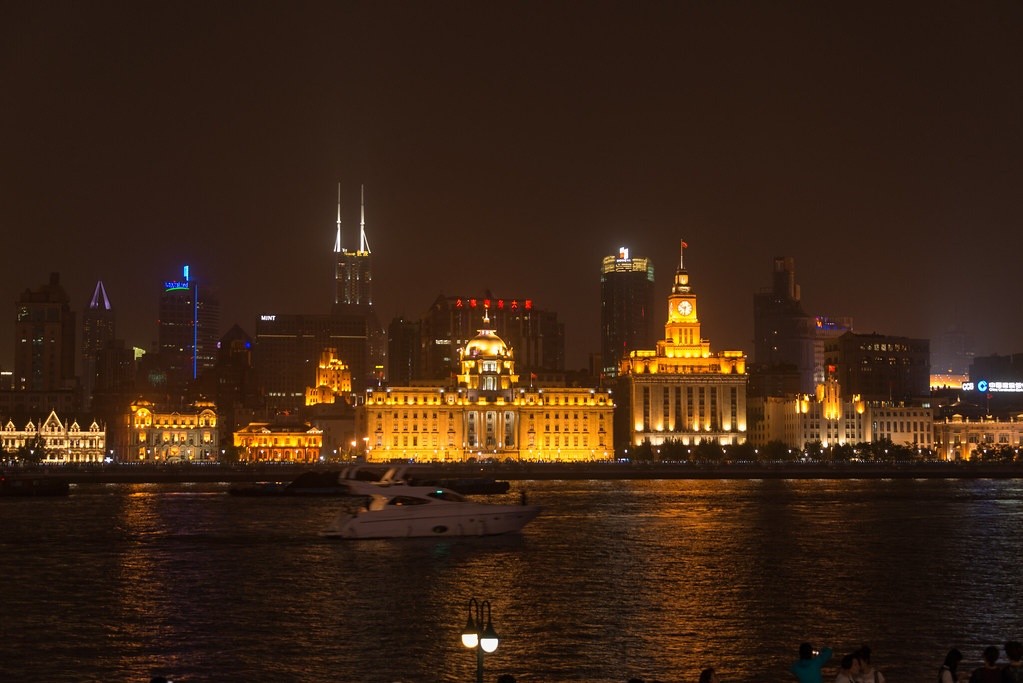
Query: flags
682,239,688,249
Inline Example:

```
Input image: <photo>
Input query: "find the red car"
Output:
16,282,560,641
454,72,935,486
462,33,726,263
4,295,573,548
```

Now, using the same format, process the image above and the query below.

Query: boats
316,464,543,538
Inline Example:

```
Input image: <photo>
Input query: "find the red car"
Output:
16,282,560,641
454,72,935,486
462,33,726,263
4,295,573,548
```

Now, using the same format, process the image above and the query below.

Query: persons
835,645,886,683
969,640,1023,683
699,668,720,683
788,642,832,683
937,648,968,683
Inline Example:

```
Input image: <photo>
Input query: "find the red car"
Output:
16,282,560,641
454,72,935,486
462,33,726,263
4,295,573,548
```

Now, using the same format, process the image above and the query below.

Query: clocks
677,300,692,317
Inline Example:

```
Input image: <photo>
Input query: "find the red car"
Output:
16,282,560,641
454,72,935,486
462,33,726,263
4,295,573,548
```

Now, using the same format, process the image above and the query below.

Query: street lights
462,598,499,682
